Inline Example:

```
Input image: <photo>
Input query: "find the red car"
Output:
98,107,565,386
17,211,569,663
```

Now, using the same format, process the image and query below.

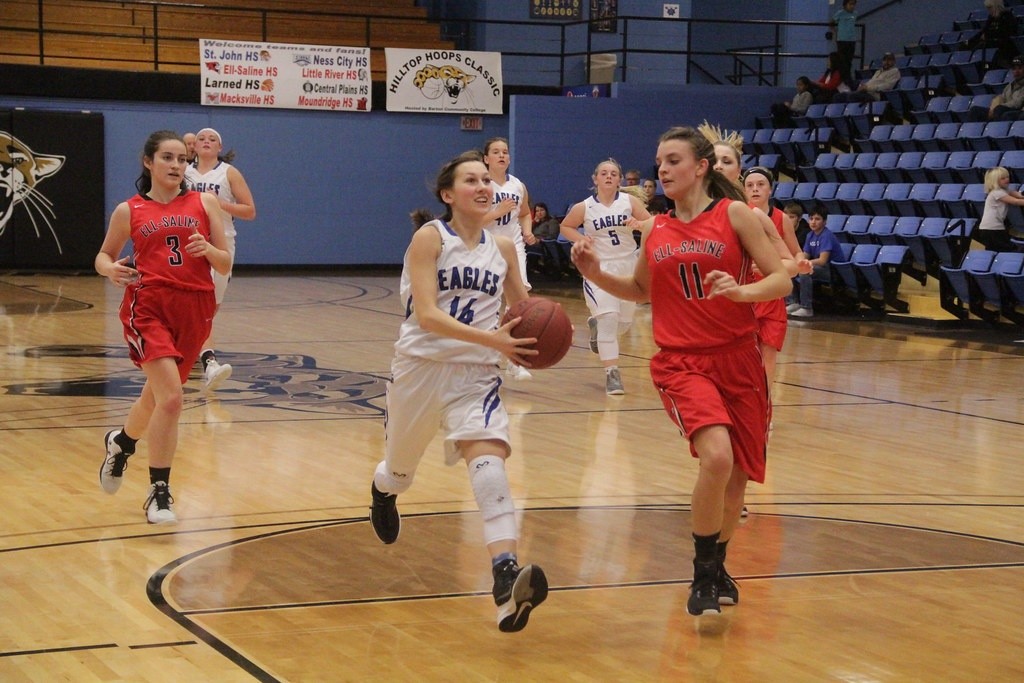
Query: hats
882,52,895,59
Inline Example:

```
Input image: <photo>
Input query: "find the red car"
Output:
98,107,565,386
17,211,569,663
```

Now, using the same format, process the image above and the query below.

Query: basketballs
500,297,572,370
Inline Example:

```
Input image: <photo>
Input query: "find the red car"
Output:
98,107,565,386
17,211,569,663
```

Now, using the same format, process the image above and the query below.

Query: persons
743,165,815,398
976,165,1024,251
530,202,560,240
569,128,791,619
786,206,844,317
180,127,256,390
369,151,574,633
829,0,858,82
988,56,1024,117
479,135,540,380
974,0,1019,52
770,76,813,116
696,120,801,278
806,51,844,105
782,203,812,305
859,52,901,102
624,169,642,186
558,155,653,396
91,129,231,525
642,177,670,218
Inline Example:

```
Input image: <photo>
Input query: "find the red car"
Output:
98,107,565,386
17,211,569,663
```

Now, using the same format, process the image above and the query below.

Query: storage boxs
583,53,617,84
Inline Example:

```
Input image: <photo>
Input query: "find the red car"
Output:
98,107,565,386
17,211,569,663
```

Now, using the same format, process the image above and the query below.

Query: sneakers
494,561,549,632
791,308,814,317
717,564,739,606
368,479,400,544
204,359,232,388
786,303,802,315
99,430,135,494
606,368,625,395
143,480,175,524
686,577,722,617
506,360,531,381
587,317,599,354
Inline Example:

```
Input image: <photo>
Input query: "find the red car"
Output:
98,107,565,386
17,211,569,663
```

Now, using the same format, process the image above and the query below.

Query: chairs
526,10,1024,330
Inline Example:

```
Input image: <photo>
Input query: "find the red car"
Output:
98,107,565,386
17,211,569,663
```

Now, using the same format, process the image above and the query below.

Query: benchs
0,0,456,83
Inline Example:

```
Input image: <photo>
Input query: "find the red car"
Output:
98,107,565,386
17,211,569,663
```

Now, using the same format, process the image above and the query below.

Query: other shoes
740,507,749,517
